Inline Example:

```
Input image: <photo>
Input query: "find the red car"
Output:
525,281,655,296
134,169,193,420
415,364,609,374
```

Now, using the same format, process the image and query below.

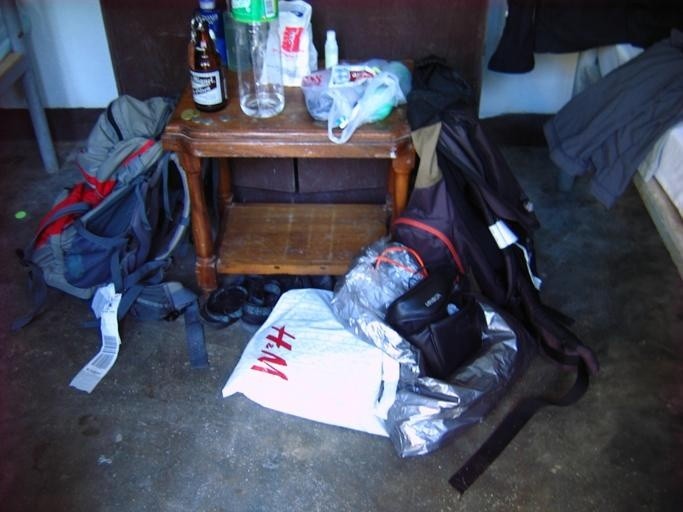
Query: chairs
0,1,60,181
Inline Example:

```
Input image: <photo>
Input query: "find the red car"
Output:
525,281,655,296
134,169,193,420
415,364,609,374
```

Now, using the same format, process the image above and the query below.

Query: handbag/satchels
390,52,540,309
385,265,489,380
221,288,401,438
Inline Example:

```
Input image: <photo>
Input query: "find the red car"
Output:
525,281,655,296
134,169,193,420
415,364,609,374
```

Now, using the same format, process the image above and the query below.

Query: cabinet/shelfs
159,56,419,312
99,0,493,202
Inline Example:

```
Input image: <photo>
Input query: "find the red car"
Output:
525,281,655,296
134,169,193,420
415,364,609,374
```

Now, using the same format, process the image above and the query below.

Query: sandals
198,272,303,333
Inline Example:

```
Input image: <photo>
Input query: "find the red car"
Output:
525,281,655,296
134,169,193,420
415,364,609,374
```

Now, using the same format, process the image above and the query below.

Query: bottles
187,18,231,113
192,0,228,66
229,1,286,119
324,29,338,68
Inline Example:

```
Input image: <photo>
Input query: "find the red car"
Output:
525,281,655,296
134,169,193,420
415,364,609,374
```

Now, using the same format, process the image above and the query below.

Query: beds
572,45,683,292
